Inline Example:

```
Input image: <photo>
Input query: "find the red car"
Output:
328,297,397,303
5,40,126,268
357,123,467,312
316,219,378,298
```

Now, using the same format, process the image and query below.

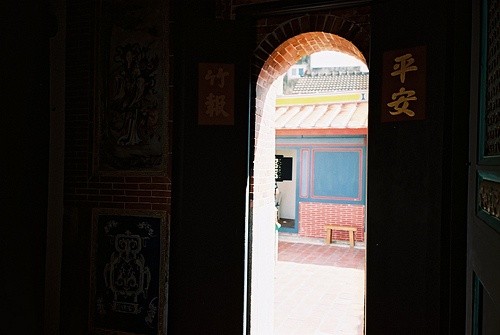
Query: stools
326,224,357,248
